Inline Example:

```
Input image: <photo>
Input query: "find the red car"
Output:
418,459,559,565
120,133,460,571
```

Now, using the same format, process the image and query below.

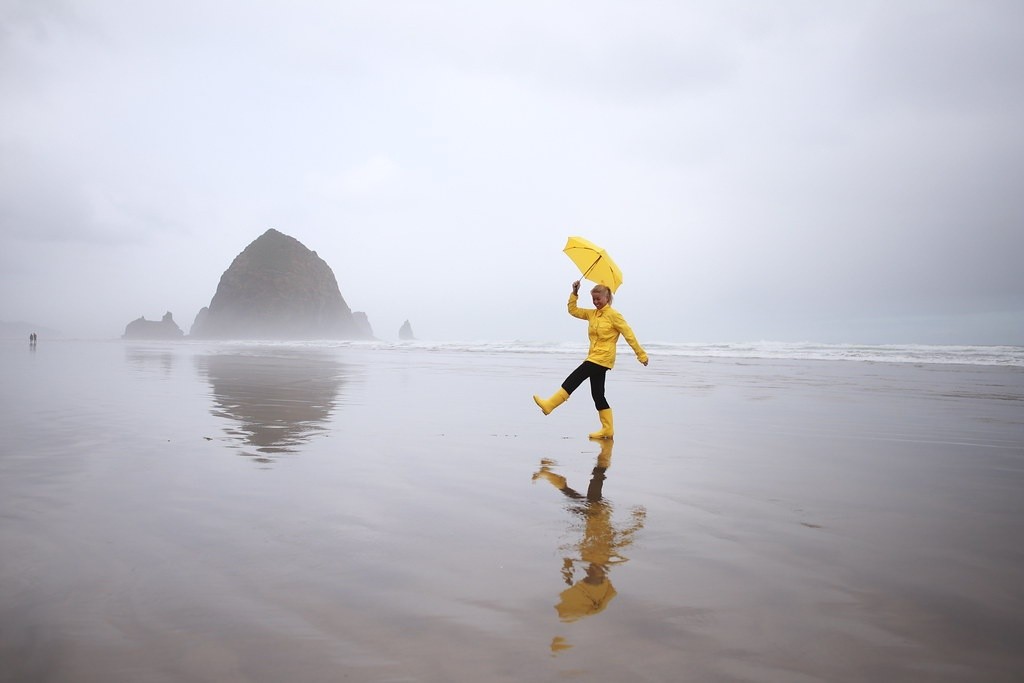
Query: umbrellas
562,236,623,294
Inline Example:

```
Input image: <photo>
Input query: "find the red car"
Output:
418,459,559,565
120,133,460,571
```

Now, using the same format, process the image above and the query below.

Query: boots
534,468,566,489
589,408,613,438
590,438,614,462
534,387,569,415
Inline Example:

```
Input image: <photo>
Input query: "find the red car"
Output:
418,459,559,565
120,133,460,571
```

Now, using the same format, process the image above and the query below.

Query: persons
30,333,36,342
533,280,648,439
